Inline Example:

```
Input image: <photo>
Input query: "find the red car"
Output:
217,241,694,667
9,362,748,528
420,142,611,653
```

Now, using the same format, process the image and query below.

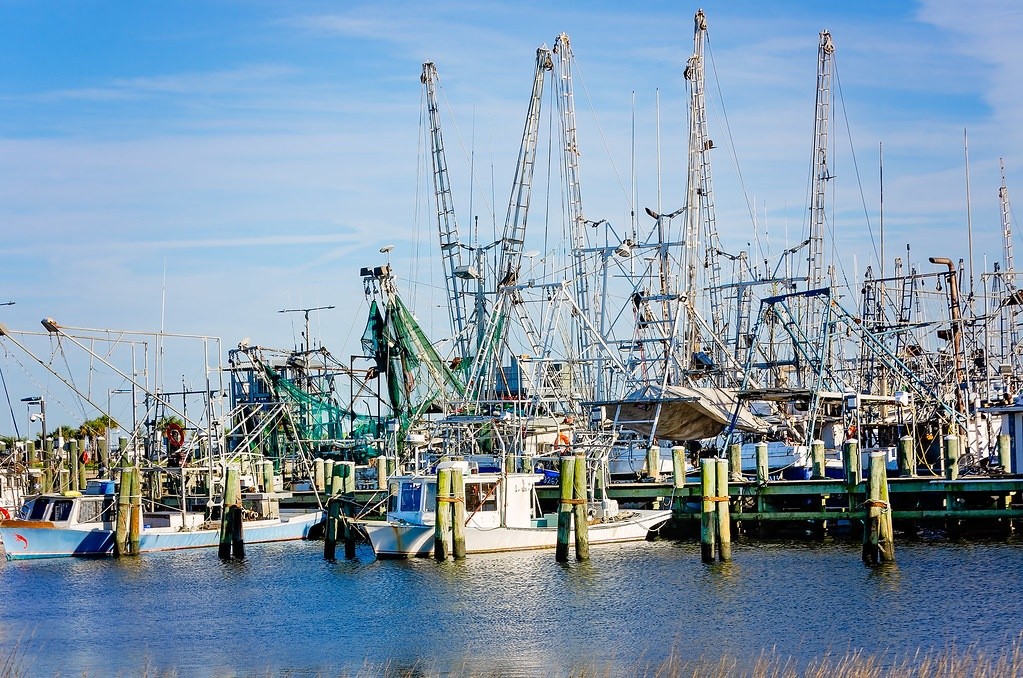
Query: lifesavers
847,426,856,439
553,435,570,456
166,423,184,447
82,451,90,465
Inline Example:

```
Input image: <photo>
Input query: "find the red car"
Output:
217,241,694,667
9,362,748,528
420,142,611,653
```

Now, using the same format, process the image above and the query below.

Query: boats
0,12,1023,557
